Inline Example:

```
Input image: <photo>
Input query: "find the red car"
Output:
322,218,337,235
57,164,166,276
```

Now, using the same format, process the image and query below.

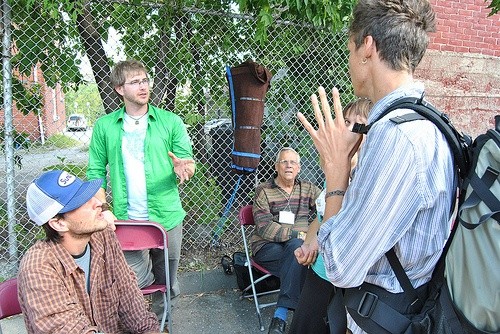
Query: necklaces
124,110,149,125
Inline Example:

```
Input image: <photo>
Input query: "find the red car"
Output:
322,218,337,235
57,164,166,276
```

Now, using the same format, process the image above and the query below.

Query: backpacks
343,97,500,334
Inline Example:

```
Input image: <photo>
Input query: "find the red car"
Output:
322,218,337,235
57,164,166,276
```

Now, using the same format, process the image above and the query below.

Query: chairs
0,278,23,334
113,220,173,334
239,205,281,332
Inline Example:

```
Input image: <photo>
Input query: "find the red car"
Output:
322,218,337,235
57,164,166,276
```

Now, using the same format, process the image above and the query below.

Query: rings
183,171,188,177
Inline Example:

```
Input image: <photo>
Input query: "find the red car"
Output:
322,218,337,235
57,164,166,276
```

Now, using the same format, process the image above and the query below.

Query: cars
67,115,86,131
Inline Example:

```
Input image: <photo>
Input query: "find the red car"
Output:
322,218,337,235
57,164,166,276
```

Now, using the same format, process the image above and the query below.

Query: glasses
280,160,299,165
126,78,149,85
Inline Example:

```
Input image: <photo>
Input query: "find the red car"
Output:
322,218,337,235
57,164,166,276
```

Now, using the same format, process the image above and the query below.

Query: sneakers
268,317,286,334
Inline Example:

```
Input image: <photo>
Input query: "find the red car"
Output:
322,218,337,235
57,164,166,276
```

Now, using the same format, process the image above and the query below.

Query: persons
16,170,160,334
250,147,323,334
297,0,460,334
86,60,196,334
288,99,374,334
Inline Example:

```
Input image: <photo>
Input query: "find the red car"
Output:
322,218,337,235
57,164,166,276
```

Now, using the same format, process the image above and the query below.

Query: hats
26,170,102,227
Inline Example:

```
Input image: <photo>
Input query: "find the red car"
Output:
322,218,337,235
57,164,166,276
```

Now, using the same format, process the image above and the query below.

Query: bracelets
325,190,345,202
